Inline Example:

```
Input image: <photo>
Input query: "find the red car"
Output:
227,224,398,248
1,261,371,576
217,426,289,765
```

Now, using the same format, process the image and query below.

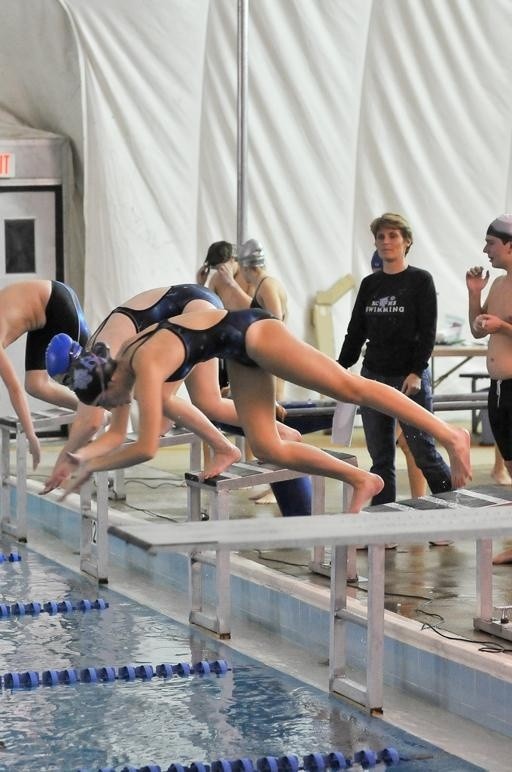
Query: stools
458,372,491,434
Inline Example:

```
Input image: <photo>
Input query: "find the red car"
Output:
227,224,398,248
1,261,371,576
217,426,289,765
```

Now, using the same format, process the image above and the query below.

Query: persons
218,238,289,424
361,244,432,503
0,270,91,473
219,259,255,401
468,211,512,491
55,307,476,516
35,280,305,497
333,206,457,510
196,241,234,399
488,433,512,487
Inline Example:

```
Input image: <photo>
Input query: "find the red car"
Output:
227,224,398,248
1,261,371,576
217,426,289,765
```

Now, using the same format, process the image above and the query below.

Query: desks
431,345,488,394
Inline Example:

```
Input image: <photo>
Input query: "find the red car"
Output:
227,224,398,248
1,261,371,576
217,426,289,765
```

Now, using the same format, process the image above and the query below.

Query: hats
238,239,264,268
487,214,512,240
46,334,114,405
